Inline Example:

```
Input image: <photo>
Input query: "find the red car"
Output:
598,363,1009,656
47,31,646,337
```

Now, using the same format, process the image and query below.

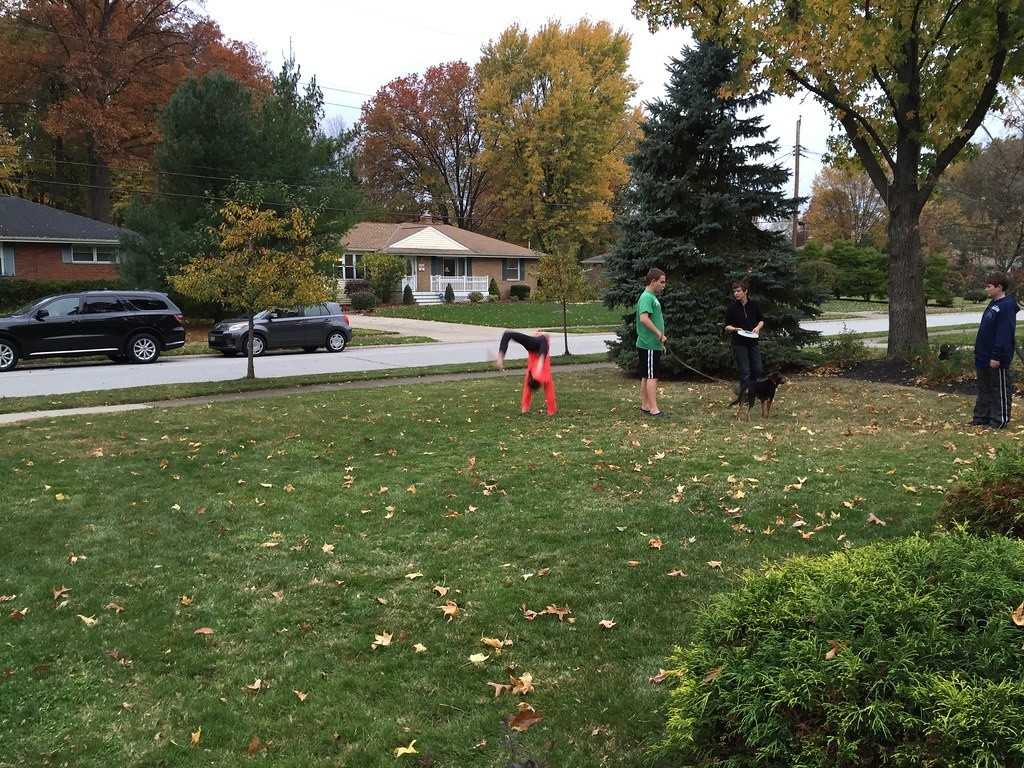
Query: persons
489,330,556,415
636,268,668,417
966,272,1020,429
725,281,764,406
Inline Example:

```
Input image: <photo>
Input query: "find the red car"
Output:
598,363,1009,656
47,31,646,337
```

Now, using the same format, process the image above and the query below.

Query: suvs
0,288,186,373
207,300,353,357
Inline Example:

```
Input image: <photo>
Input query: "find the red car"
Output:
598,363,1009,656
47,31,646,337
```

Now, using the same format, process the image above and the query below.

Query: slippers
641,408,650,414
649,411,666,418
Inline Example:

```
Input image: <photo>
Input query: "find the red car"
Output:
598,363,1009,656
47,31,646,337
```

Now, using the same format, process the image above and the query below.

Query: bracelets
734,328,737,331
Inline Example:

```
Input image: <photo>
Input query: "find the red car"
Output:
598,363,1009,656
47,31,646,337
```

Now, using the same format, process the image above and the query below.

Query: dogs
728,372,785,423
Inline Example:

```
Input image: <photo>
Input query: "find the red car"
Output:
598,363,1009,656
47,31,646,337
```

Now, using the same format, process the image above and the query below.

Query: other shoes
982,424,992,430
966,421,976,426
